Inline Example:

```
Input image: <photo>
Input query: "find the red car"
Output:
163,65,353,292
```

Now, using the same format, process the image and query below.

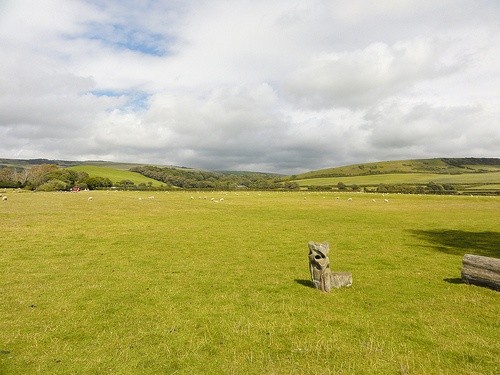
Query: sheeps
0,186,499,215
